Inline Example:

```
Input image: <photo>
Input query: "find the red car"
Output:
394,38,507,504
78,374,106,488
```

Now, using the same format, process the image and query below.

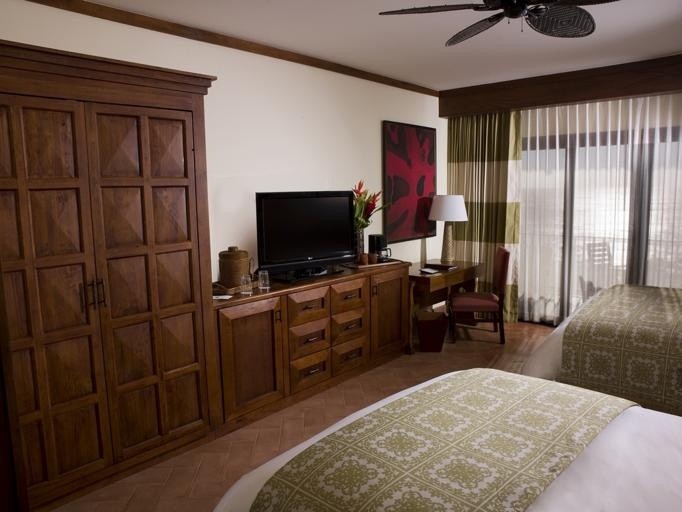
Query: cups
358,253,368,265
367,253,378,263
258,270,270,290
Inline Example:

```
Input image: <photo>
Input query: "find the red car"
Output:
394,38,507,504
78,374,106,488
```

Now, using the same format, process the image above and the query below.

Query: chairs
450,246,512,339
586,237,627,274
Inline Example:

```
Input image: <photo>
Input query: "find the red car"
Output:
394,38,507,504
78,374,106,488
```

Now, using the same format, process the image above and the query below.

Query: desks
403,257,484,350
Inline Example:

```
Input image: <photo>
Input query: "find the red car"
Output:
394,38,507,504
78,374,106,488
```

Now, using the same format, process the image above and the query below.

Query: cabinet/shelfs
0,38,224,510
215,254,414,436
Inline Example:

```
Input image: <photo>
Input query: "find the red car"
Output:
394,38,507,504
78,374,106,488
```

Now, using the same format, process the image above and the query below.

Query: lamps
427,192,469,261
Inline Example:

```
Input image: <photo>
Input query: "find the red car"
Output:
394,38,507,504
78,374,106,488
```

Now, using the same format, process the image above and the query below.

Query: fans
377,0,623,42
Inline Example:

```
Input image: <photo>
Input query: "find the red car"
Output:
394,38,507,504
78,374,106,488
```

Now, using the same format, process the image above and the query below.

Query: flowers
351,180,390,227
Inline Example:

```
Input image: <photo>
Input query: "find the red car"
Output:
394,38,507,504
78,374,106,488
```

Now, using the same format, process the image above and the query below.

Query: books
424,261,457,272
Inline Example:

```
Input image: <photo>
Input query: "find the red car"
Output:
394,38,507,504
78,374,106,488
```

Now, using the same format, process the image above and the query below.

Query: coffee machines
368,234,392,263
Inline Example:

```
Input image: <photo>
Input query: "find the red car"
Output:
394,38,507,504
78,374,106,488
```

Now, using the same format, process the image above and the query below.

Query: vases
355,227,364,264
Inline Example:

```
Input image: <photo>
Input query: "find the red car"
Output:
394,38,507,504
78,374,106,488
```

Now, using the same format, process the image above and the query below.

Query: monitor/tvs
255,191,358,284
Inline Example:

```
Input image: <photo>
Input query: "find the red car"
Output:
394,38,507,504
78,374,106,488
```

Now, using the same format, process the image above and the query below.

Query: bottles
241,274,253,295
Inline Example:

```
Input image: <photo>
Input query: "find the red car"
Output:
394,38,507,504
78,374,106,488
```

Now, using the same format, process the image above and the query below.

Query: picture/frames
381,120,437,244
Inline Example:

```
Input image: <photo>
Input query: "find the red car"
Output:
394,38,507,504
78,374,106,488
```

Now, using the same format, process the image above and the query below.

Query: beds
211,363,681,512
520,282,682,414
